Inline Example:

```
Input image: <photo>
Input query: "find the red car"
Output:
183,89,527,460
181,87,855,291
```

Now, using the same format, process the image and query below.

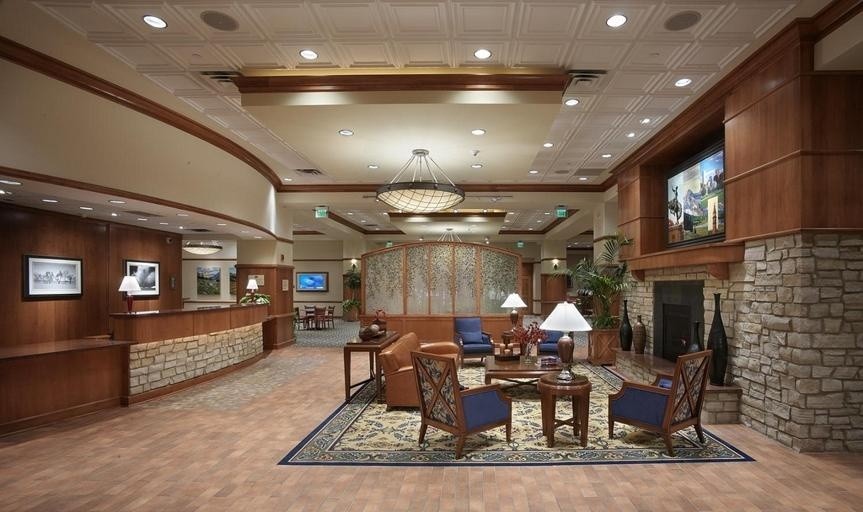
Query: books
536,354,560,367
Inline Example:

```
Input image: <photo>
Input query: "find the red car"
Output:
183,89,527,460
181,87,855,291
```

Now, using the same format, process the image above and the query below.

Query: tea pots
359,319,376,339
373,308,387,335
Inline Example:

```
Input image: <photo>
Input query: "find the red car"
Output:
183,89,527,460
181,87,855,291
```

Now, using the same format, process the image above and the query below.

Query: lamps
182,244,223,255
551,259,560,270
500,293,528,328
539,301,593,380
376,149,465,215
246,279,259,306
118,276,143,313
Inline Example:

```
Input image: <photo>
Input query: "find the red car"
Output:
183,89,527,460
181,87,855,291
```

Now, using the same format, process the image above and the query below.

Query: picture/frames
123,259,160,299
23,254,84,299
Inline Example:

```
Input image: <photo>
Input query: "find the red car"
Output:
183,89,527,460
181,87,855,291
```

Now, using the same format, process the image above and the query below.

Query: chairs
454,316,496,369
608,350,714,458
378,332,459,412
409,350,512,460
294,304,335,330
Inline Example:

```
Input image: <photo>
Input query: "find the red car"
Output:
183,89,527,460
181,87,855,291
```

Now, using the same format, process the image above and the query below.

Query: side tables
501,334,513,347
343,332,400,404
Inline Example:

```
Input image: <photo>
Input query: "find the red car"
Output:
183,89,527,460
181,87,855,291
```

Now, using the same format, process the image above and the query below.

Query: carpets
277,371,757,467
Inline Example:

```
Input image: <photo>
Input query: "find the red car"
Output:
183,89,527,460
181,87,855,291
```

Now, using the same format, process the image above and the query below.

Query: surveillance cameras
165,236,174,244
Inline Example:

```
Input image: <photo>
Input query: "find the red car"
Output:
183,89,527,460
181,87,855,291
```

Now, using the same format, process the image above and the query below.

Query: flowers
513,321,546,345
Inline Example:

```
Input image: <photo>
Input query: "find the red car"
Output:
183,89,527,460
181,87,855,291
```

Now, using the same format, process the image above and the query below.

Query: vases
632,314,647,355
687,322,702,354
706,293,728,386
529,343,537,356
620,299,633,351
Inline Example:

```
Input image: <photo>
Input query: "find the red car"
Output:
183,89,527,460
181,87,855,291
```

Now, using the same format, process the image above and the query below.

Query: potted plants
341,299,360,322
547,230,635,366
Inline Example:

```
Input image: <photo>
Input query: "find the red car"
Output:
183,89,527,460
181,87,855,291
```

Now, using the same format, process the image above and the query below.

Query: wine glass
500,331,514,354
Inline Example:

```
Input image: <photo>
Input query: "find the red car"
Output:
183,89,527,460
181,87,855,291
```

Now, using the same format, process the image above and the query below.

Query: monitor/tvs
662,137,725,251
